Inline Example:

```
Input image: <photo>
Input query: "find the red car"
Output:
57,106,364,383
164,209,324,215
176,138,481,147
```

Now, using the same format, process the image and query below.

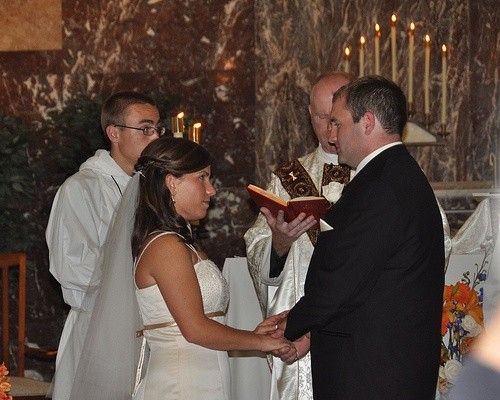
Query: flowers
0,362,13,400
435,235,494,396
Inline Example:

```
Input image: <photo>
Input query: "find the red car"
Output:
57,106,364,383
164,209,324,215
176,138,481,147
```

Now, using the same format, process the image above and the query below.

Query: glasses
114,125,166,136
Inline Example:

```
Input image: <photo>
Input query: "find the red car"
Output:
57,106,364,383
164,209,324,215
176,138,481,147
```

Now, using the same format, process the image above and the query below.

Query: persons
44,92,165,400
267,75,445,400
268,72,451,400
47,137,297,400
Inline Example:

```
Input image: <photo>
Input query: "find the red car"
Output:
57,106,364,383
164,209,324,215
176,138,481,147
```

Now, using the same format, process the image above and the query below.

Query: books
244,184,324,232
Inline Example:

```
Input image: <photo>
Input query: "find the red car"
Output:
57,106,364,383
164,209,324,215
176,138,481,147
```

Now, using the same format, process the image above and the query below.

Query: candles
408,22,415,102
391,14,398,83
344,47,349,73
374,24,380,75
173,112,185,137
424,35,431,114
187,122,202,144
441,44,447,124
359,36,366,76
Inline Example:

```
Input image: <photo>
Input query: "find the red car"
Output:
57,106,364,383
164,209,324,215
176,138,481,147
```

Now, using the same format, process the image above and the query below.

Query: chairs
0,254,54,400
